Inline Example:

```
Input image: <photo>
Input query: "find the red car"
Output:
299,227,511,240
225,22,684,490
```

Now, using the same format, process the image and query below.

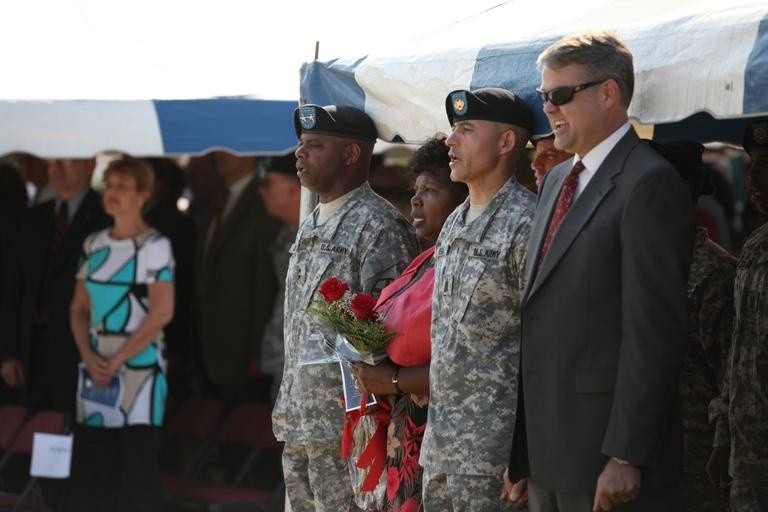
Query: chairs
1,401,76,512
175,392,282,511
113,389,226,511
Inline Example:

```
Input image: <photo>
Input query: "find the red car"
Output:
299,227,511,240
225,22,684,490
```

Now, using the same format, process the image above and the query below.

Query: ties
54,201,69,239
211,187,230,238
534,163,585,271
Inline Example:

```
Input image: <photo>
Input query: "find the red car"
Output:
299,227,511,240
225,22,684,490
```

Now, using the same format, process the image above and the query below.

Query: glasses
536,81,602,105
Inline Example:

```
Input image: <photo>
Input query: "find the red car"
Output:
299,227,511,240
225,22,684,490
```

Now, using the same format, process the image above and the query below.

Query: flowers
315,274,392,357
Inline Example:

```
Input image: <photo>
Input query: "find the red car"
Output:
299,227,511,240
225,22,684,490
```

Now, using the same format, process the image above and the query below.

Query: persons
337,129,472,510
270,106,415,510
415,85,537,510
507,28,677,510
0,147,767,512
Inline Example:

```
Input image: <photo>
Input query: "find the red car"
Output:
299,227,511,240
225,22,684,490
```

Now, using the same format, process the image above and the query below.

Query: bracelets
612,456,630,465
390,365,405,396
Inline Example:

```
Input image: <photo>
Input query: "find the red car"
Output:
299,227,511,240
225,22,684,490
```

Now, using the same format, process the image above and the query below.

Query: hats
293,104,377,140
261,152,296,173
661,140,716,194
742,119,768,155
445,87,530,129
